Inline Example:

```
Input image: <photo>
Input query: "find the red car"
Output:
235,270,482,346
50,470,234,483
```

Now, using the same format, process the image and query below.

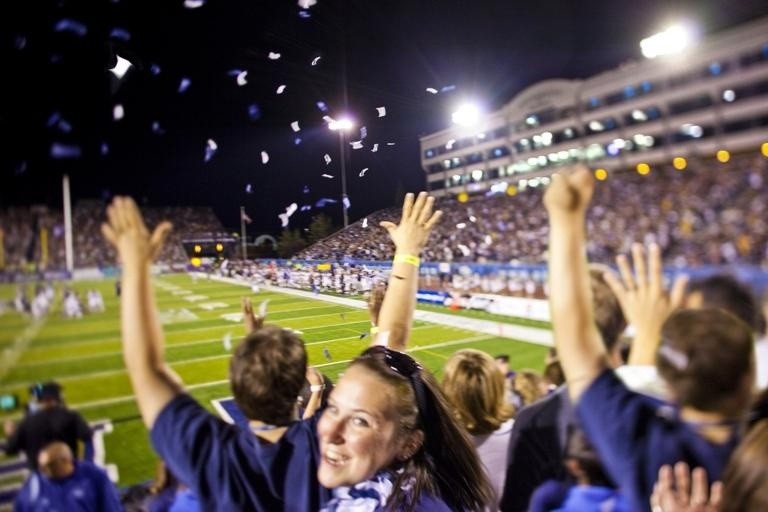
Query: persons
0,155,767,512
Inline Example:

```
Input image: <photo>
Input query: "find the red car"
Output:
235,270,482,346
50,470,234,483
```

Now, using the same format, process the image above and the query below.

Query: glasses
362,344,422,409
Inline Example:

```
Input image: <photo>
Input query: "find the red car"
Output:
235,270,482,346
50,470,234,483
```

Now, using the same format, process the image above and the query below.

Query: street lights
327,112,357,224
